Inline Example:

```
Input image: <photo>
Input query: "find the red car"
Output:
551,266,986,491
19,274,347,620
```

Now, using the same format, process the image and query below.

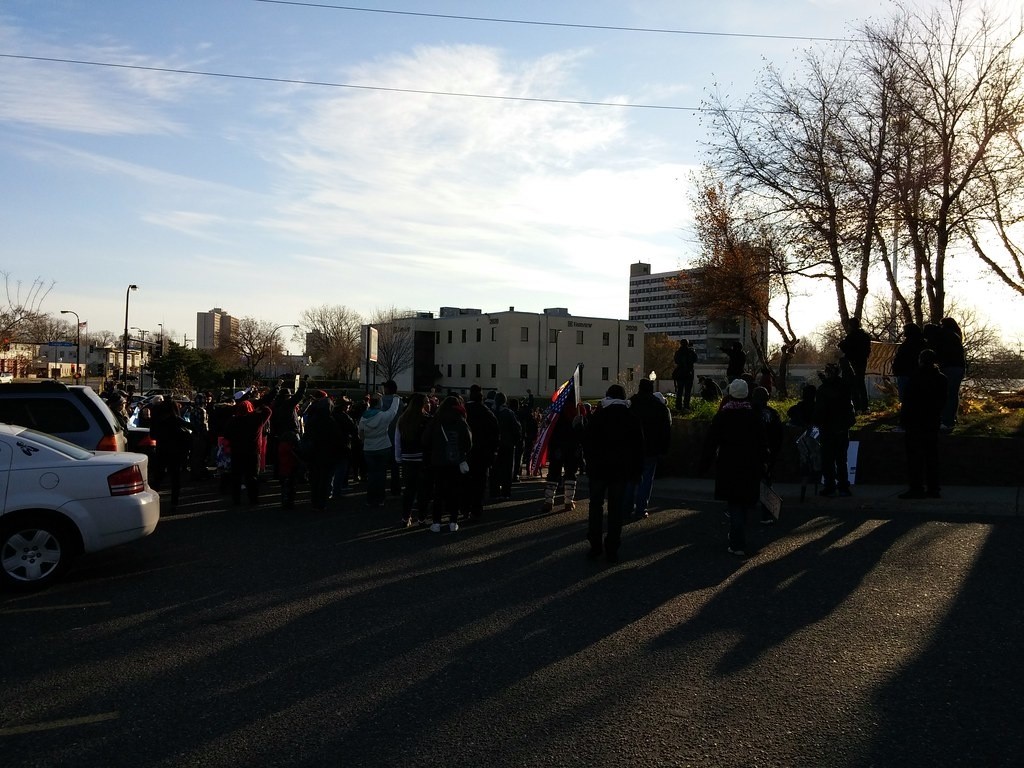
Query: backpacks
442,431,461,464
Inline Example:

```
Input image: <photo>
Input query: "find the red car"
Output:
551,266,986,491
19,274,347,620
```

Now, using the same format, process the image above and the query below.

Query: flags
527,365,581,477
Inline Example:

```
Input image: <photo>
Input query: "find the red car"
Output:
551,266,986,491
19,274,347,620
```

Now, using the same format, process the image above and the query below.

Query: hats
698,377,706,384
332,397,353,408
729,379,748,399
236,400,256,416
605,384,626,400
445,396,460,407
276,388,294,396
109,393,124,408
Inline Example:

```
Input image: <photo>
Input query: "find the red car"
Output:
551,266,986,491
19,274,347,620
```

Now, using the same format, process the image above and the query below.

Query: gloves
304,375,309,381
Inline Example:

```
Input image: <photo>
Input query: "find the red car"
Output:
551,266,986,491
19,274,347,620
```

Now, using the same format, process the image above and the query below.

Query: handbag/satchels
573,445,591,472
672,367,680,392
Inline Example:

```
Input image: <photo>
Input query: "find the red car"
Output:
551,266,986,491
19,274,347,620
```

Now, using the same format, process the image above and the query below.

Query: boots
564,480,577,512
800,485,807,503
402,517,412,526
282,486,296,510
390,479,402,496
513,474,520,482
543,482,559,513
585,530,604,560
604,537,622,563
418,519,426,526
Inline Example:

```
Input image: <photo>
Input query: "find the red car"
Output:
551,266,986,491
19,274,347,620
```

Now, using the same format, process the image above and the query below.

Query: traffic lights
70,364,75,373
3,337,10,352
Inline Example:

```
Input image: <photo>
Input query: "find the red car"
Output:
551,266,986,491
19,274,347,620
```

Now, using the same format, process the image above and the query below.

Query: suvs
0,380,125,453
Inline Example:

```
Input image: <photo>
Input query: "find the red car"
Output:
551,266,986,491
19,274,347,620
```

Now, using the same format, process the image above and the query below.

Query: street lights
158,323,163,357
123,285,139,389
61,311,86,385
269,325,300,372
130,326,149,396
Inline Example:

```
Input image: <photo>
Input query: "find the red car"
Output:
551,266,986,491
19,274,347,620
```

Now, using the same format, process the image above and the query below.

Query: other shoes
722,511,731,521
760,517,773,524
898,488,926,499
819,489,836,498
728,532,732,542
728,545,745,555
635,511,649,519
925,489,941,498
839,491,851,496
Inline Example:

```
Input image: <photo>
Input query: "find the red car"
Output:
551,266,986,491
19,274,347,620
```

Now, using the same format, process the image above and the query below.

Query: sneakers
429,524,440,532
448,523,459,532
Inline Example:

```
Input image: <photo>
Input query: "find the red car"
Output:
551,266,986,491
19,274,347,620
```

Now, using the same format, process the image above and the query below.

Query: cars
0,420,159,590
0,373,14,383
112,373,137,381
129,389,190,409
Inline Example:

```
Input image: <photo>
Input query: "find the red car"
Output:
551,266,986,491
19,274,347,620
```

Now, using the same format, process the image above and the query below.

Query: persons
100,379,589,539
671,338,698,411
627,379,672,518
711,317,964,555
580,384,643,559
696,376,723,402
